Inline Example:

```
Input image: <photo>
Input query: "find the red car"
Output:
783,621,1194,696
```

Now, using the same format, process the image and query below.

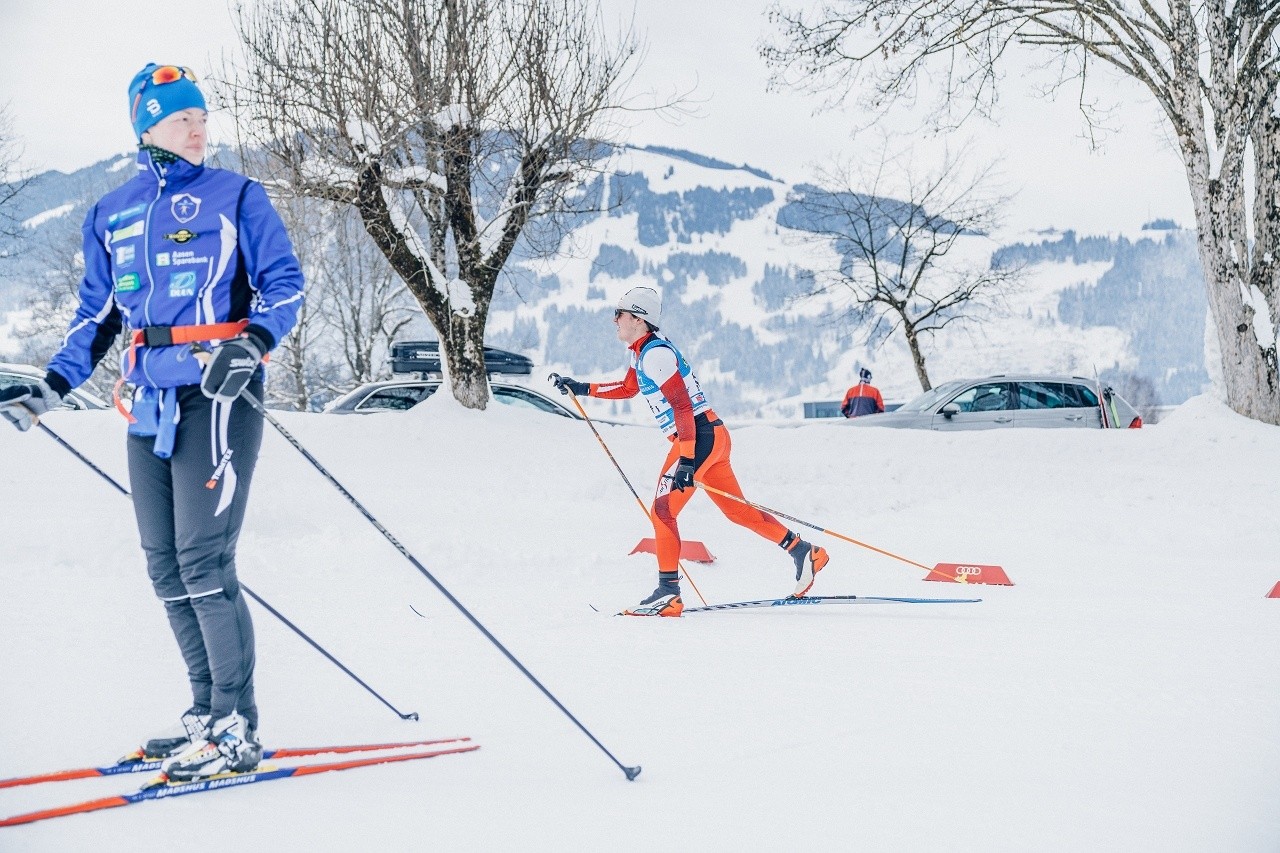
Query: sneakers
626,585,684,616
791,540,829,598
144,706,212,759
161,714,267,782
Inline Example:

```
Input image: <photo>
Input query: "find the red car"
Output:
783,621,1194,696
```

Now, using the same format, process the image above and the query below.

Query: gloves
554,377,590,396
0,378,63,433
200,331,268,403
672,456,695,492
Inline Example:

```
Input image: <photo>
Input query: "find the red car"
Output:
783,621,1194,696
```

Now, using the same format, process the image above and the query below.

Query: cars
832,373,1143,430
0,362,113,411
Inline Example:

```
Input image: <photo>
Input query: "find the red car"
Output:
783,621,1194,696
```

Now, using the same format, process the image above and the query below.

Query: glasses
615,309,648,319
132,65,197,122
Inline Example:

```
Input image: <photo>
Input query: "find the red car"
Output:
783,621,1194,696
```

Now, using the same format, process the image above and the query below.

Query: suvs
318,338,585,420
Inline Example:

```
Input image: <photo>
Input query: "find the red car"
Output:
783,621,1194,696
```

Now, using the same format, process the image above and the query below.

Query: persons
0,64,301,783
552,287,829,617
842,369,884,418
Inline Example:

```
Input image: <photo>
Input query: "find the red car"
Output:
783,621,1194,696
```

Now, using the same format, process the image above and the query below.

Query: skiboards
0,735,481,831
586,593,985,620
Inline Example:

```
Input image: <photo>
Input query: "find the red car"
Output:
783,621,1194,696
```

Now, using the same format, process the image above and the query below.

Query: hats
128,63,208,144
618,286,662,327
860,368,872,383
